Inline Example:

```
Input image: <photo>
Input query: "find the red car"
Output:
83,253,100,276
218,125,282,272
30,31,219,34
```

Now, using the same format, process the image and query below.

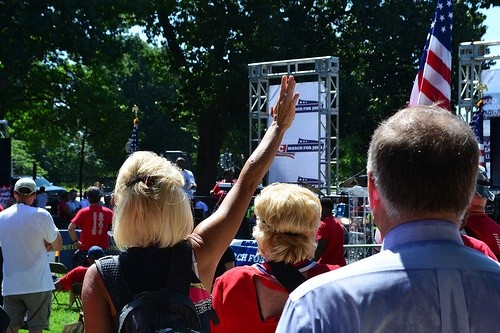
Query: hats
14,178,37,195
476,165,495,201
88,246,106,260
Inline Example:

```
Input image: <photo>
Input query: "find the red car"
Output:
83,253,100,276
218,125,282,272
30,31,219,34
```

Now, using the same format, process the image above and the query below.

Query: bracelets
73,238,78,242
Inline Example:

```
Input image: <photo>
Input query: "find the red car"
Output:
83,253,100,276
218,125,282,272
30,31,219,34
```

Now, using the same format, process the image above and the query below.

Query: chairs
49,263,82,313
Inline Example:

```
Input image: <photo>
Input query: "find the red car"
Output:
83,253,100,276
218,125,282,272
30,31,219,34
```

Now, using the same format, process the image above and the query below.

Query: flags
0,185,11,212
130,116,139,153
407,0,456,115
469,113,488,176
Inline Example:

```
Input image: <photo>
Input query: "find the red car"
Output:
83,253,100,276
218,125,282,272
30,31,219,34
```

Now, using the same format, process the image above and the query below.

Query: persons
57,190,113,229
209,182,342,333
68,185,113,250
0,178,63,333
36,187,49,209
315,199,347,267
175,157,197,221
82,74,299,333
275,106,500,333
460,166,499,262
54,245,107,292
211,168,238,202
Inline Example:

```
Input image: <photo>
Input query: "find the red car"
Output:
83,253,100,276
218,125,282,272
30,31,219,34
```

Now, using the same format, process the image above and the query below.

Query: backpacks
94,240,203,333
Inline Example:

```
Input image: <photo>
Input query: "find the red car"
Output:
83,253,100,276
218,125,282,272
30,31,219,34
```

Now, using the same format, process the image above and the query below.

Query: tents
35,177,67,195
